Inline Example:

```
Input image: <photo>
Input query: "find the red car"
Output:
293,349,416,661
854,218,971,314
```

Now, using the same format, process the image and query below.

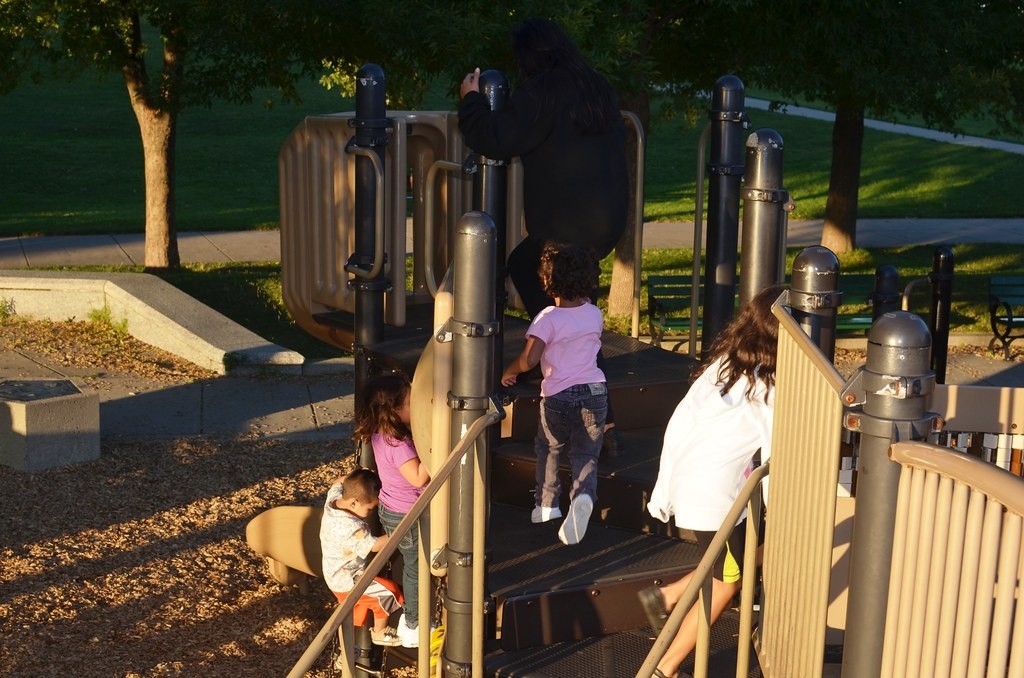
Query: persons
501,238,608,546
351,374,436,648
319,467,389,671
637,284,790,678
456,18,630,459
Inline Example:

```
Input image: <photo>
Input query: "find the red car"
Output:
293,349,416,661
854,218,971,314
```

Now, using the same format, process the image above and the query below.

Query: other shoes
650,668,692,678
637,585,669,638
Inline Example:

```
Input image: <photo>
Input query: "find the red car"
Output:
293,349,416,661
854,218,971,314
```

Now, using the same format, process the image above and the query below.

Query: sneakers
369,624,401,646
531,501,562,523
396,613,438,648
336,652,343,671
558,492,593,545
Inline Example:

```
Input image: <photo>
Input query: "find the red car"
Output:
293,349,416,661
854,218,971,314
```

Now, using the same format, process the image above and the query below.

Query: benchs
988,275,1024,360
647,274,878,348
